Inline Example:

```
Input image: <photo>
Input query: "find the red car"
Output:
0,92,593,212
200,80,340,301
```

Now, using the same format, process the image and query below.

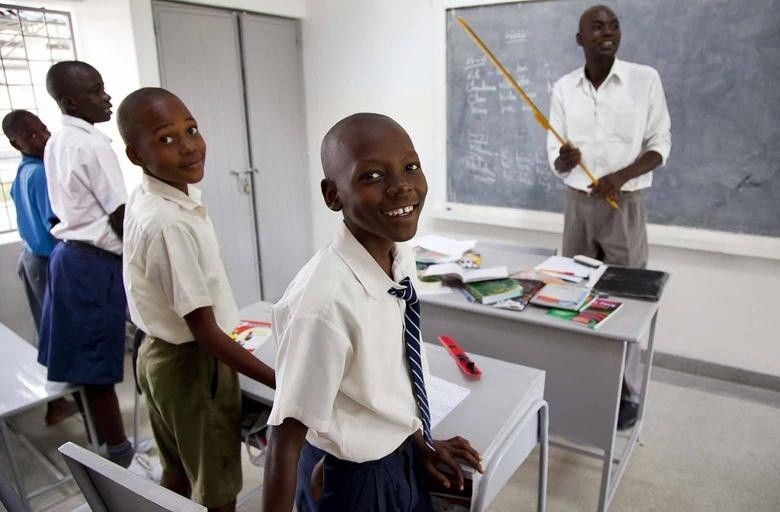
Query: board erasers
574,254,603,268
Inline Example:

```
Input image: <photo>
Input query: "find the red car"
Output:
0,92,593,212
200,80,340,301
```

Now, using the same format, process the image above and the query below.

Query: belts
570,188,629,196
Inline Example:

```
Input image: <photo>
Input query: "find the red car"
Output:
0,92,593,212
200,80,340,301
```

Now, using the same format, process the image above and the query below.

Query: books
409,234,623,331
232,318,274,351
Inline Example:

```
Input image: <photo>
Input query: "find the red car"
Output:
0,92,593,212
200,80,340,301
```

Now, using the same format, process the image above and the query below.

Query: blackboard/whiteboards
445,0,780,259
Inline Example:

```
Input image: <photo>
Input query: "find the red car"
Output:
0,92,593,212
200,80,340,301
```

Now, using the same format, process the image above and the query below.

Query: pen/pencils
457,286,473,302
572,295,621,331
539,266,574,276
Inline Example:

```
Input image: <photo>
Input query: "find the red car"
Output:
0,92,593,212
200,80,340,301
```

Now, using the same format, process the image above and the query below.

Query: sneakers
87,436,164,484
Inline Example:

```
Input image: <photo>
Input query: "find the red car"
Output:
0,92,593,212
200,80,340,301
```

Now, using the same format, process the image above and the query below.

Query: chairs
55,433,215,512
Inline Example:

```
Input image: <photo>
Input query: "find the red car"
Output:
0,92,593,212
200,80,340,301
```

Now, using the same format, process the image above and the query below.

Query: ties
388,278,436,452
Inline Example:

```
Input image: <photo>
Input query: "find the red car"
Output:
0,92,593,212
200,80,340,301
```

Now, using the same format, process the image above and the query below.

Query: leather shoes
617,399,639,431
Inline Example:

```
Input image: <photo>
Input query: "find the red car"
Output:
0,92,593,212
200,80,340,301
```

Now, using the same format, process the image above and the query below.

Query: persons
543,5,674,432
35,60,147,469
260,113,485,511
115,86,277,511
2,108,81,426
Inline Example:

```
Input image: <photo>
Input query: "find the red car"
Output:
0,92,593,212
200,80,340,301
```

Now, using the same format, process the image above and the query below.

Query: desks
233,249,670,512
0,322,101,511
